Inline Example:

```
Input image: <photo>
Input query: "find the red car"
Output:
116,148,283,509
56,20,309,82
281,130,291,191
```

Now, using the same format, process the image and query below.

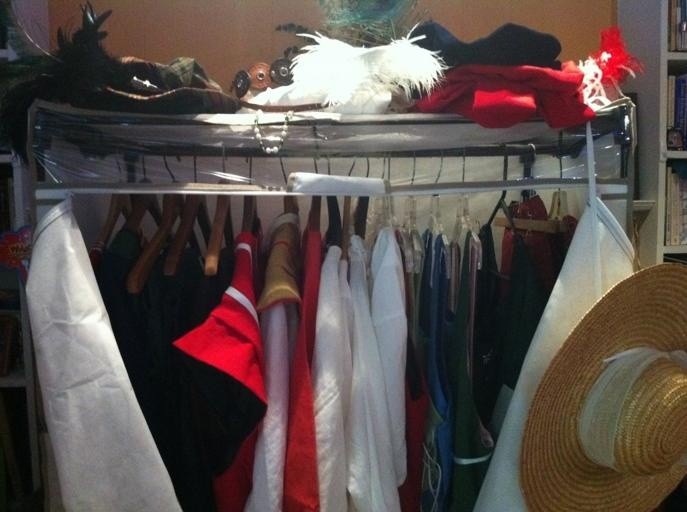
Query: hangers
88,143,571,297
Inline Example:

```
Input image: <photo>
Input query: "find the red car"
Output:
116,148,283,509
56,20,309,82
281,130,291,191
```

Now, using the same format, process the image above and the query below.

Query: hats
521,262,685,512
407,20,561,74
0,1,246,158
228,1,447,152
410,25,643,131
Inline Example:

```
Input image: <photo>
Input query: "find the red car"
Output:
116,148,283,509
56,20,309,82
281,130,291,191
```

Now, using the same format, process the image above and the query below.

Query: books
664,1,686,246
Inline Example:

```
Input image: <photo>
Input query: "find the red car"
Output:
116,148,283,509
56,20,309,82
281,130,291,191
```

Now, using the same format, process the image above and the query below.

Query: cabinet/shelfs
616,1,686,276
0,149,50,512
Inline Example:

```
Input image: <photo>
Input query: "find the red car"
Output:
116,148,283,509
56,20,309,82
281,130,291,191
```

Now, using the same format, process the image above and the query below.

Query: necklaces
254,106,294,155
